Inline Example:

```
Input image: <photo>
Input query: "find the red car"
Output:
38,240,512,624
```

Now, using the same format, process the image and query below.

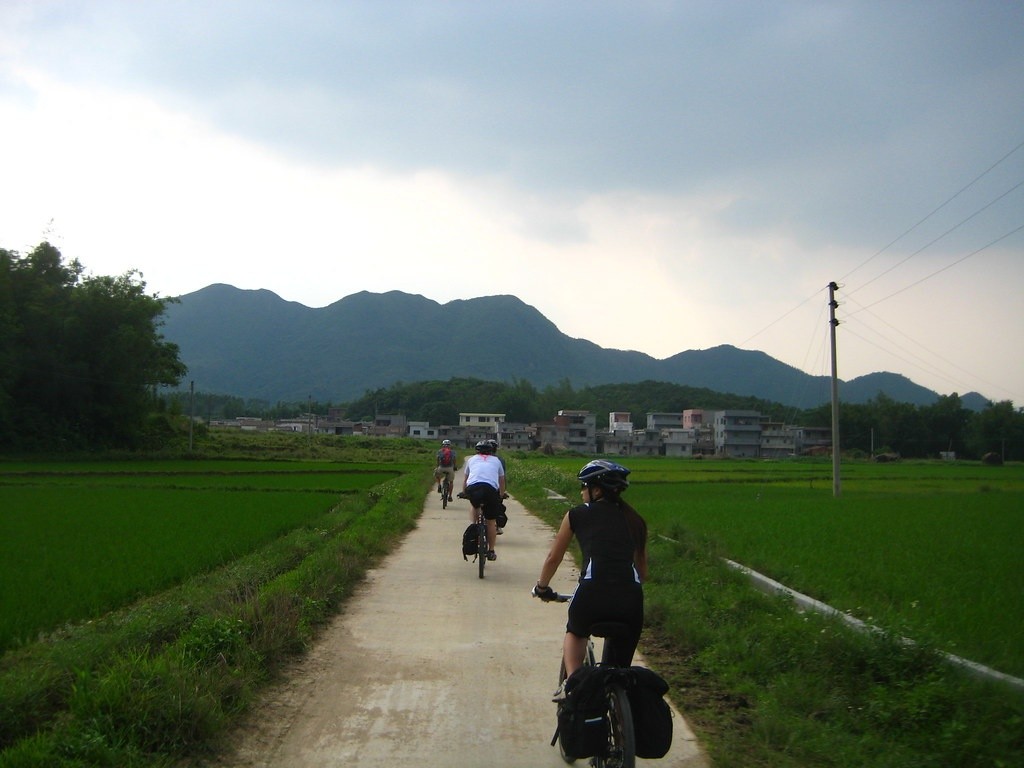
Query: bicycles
531,581,636,767
433,467,459,509
458,494,509,578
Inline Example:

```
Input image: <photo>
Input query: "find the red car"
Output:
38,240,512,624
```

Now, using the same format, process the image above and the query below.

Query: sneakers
448,497,453,501
496,527,504,535
437,485,442,493
487,552,497,561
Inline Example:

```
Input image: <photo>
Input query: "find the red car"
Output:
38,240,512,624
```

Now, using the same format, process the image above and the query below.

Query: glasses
581,477,601,491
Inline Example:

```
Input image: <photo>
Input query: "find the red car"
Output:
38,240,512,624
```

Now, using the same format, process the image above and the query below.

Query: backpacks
622,665,673,760
462,523,480,563
552,664,611,759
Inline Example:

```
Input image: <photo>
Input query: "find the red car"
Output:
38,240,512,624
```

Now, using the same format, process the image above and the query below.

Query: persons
463,439,506,561
434,439,457,502
531,460,649,702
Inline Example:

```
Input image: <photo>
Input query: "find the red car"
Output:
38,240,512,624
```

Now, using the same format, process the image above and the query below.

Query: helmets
475,440,493,453
488,439,499,453
577,458,631,503
442,440,451,447
